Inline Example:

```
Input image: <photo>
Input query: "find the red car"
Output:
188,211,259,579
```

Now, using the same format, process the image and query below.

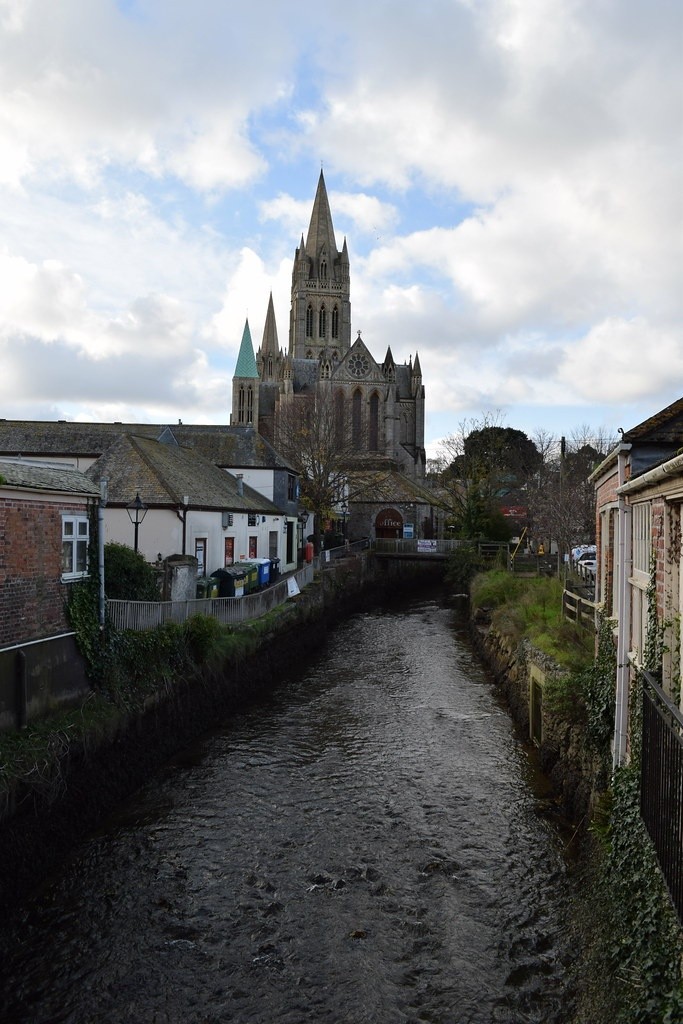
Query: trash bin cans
307,534,325,556
196,557,281,609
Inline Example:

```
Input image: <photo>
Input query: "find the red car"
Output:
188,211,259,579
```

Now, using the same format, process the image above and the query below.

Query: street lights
125,492,150,553
300,508,310,569
341,503,348,545
